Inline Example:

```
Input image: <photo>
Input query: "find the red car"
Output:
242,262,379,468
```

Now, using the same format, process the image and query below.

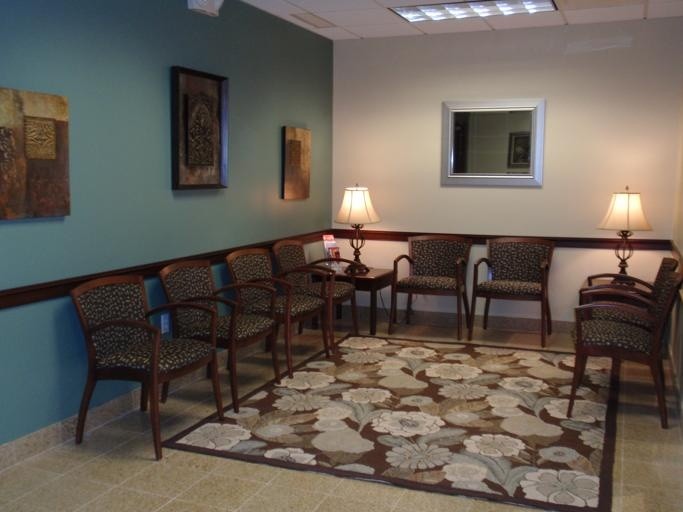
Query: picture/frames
171,63,229,192
506,131,531,169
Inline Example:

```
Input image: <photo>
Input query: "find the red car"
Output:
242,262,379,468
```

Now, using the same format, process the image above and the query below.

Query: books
322,233,341,273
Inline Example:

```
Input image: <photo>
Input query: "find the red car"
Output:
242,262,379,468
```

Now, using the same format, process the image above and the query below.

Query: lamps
593,184,654,285
333,181,384,274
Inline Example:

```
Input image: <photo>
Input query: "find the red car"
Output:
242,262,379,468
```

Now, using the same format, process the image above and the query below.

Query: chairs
389,234,472,341
68,275,226,457
471,238,556,349
158,255,281,414
564,255,681,432
225,243,331,380
271,238,362,351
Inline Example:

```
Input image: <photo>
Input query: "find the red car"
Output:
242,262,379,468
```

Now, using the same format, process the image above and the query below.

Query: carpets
162,331,623,512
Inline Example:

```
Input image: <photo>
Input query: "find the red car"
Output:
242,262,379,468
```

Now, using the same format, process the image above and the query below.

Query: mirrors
441,97,547,189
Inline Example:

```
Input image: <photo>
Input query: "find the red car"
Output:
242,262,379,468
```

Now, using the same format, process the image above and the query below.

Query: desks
321,259,397,335
